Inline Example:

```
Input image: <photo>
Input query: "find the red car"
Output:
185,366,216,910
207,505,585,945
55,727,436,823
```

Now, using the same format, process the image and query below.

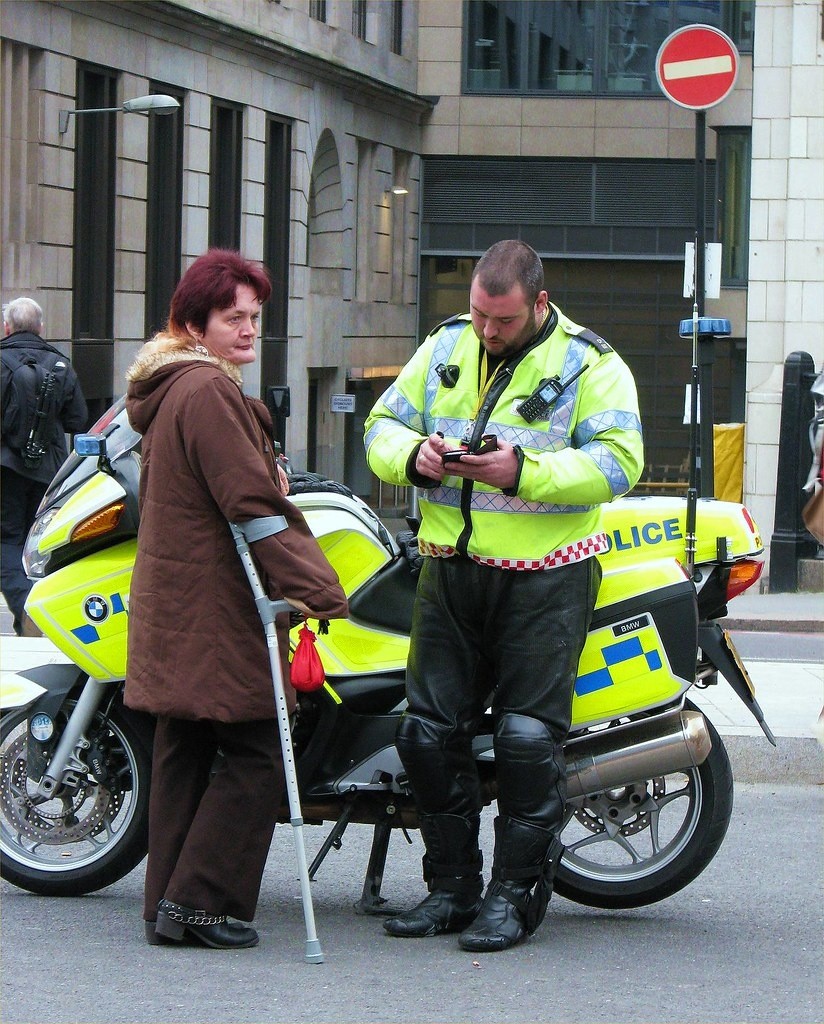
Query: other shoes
19,604,43,637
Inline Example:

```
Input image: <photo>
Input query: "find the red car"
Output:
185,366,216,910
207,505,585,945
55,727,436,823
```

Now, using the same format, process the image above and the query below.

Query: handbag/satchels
801,481,824,545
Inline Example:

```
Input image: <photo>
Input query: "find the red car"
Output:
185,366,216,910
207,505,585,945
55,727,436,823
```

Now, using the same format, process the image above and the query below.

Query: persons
0,297,89,636
126,253,351,952
360,238,647,952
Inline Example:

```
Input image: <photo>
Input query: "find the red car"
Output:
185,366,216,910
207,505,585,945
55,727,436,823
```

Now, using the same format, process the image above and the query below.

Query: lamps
59,94,181,133
384,185,408,195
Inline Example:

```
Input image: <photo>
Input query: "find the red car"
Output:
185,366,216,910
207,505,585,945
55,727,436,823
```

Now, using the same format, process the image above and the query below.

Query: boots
381,813,487,938
458,815,565,952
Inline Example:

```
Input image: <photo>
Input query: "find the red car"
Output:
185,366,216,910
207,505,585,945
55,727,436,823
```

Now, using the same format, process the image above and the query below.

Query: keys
316,617,330,636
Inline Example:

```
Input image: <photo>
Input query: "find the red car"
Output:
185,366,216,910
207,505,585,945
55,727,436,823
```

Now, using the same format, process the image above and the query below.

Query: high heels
155,900,260,949
145,914,245,947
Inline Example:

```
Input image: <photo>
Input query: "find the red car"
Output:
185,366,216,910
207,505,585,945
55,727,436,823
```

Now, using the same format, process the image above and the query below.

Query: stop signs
655,24,740,112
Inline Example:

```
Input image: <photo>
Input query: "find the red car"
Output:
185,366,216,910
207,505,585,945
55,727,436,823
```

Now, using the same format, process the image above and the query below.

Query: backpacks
0,349,70,470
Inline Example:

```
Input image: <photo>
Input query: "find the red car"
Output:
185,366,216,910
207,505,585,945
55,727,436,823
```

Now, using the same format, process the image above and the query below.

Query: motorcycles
0,393,779,918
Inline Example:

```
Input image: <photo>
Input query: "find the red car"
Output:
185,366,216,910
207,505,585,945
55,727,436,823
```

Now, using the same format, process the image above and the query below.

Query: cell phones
444,450,472,471
